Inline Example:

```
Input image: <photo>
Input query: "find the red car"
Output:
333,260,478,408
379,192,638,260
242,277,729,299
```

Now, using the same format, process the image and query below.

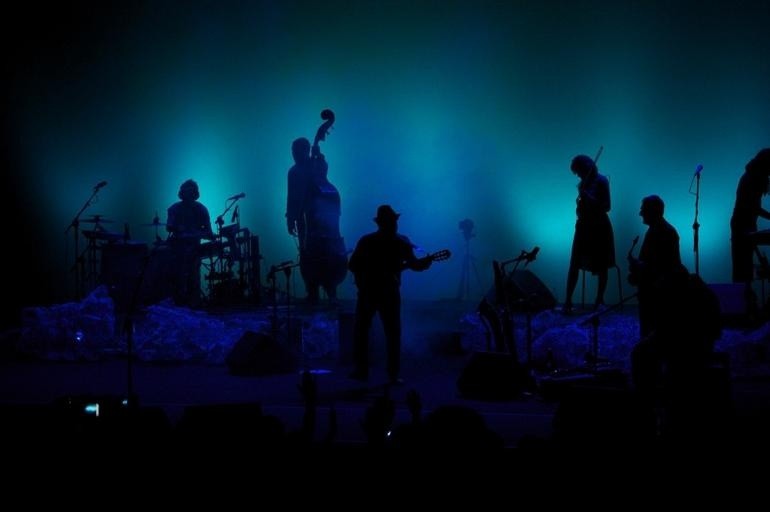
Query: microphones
694,164,703,176
96,181,107,188
525,247,539,265
230,193,245,199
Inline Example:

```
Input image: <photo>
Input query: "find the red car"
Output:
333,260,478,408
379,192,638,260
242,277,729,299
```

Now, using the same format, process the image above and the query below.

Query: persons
287,140,338,305
348,205,430,391
561,155,616,314
731,148,770,282
168,182,216,235
628,196,679,340
263,372,423,443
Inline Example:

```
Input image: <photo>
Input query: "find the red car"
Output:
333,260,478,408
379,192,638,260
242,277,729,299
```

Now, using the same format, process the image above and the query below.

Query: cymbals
142,223,166,225
81,219,111,223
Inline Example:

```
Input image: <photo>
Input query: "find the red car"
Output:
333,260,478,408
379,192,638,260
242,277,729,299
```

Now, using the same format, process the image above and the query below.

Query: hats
373,205,400,221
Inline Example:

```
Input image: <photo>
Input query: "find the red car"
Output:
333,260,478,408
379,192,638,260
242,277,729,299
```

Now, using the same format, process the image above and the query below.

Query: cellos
299,110,347,291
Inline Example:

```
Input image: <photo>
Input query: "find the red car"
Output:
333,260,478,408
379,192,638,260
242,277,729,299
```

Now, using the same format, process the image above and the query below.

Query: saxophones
627,236,642,285
